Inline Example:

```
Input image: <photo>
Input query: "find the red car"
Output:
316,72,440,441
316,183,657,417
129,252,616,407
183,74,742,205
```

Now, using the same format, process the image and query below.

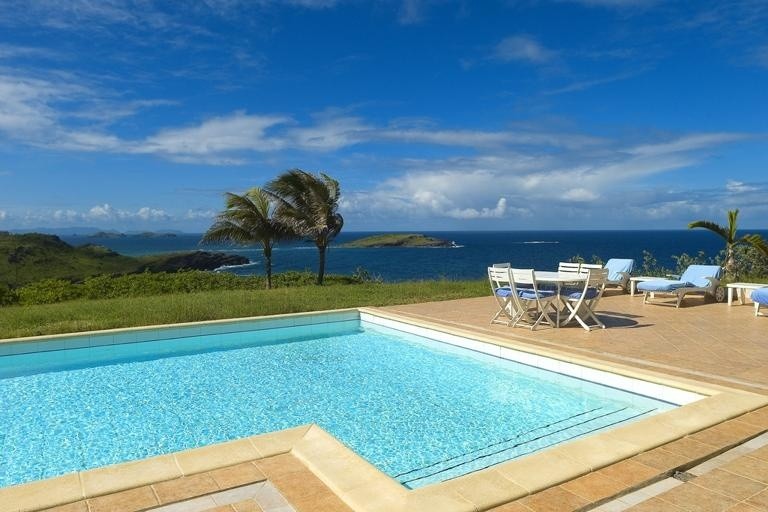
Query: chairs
486,257,768,333
